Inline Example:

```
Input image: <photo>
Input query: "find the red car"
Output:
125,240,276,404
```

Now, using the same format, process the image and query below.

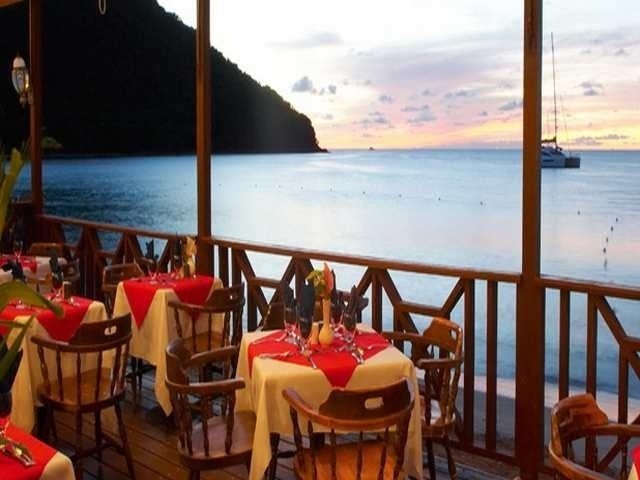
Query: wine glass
283,304,358,356
133,254,183,284
51,272,65,303
13,242,24,260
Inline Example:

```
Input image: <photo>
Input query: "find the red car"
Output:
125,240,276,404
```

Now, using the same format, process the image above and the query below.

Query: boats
538,139,581,168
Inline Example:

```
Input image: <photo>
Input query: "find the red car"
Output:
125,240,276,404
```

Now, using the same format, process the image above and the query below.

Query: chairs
546,394,639,479
